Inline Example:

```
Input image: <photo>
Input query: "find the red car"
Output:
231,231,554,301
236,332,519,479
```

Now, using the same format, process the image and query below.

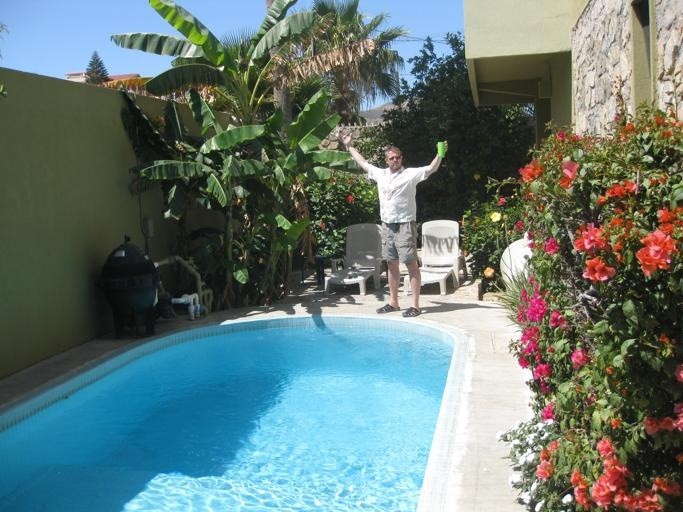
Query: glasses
388,156,401,160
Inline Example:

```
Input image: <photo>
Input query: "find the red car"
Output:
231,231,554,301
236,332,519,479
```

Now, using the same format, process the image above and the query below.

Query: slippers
376,303,400,314
403,307,422,318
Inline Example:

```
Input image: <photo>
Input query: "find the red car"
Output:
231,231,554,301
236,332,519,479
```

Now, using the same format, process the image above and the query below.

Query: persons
335,130,448,317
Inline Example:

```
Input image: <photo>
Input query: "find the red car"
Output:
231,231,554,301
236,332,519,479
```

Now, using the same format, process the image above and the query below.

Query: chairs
403,219,468,297
323,223,388,297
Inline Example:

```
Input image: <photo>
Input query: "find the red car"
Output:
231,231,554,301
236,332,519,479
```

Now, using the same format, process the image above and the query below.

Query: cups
437,141,446,159
